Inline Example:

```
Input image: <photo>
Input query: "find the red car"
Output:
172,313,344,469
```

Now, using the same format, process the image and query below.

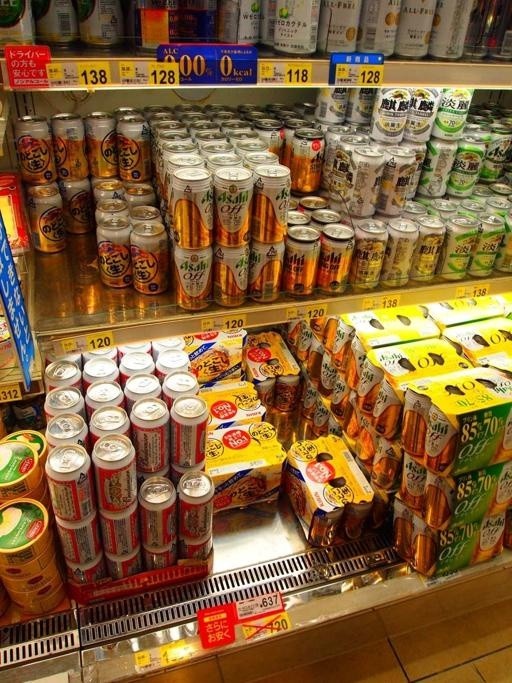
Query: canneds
0,318,460,618
0,87,512,312
0,0,512,66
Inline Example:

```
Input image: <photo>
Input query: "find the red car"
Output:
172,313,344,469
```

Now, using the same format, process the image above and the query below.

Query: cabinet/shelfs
2,0,512,682
